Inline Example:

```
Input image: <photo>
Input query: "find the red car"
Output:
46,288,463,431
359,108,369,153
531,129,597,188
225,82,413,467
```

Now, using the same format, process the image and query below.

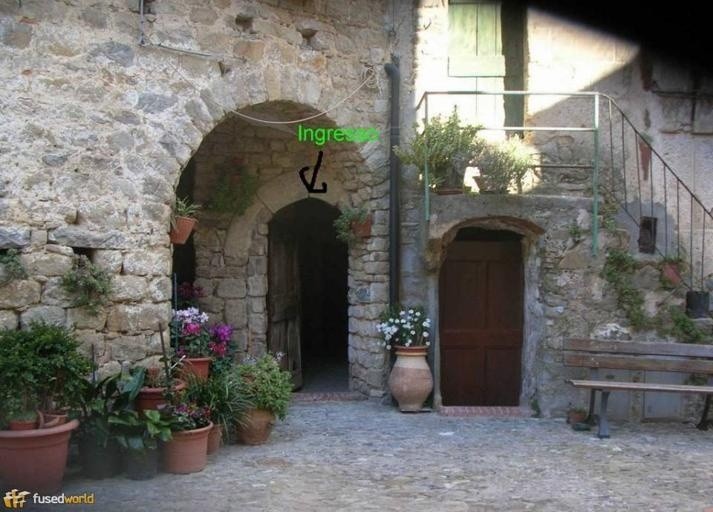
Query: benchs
557,334,712,434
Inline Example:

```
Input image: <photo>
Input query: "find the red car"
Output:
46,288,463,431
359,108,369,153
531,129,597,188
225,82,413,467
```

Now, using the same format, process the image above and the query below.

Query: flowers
369,306,433,351
141,279,294,429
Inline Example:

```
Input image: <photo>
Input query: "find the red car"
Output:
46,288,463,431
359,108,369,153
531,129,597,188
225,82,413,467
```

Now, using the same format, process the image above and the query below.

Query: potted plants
1,316,156,500
402,114,532,197
171,197,199,246
334,205,371,243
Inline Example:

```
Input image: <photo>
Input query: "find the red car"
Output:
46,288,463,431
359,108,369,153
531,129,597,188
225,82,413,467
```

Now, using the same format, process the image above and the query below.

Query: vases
387,344,434,415
135,357,276,474
